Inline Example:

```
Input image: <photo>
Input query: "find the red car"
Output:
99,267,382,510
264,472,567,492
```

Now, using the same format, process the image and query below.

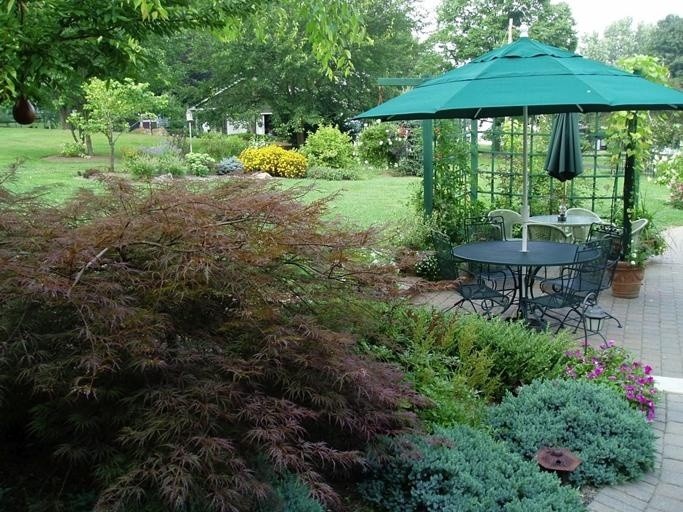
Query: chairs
430,208,651,348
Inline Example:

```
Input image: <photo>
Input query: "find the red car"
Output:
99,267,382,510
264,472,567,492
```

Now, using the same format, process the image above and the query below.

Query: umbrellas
543,112,583,198
350,25,682,299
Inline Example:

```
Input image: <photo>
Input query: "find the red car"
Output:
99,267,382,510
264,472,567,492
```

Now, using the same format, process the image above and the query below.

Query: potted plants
604,52,678,300
